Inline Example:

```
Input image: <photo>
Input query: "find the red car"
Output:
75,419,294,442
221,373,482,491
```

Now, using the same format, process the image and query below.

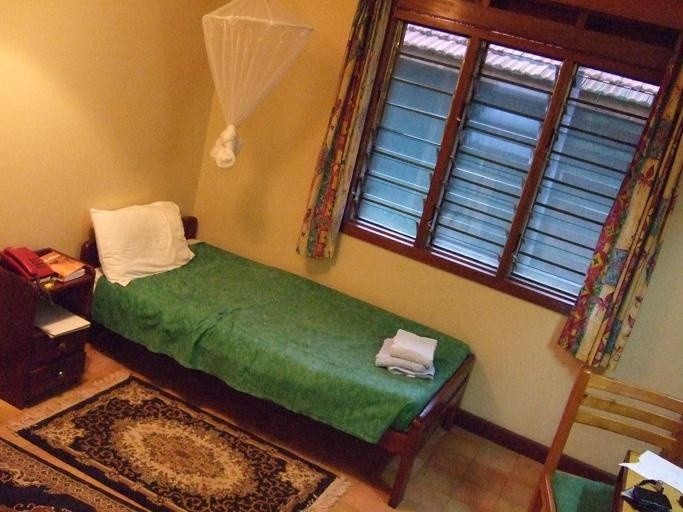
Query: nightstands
0,246,96,411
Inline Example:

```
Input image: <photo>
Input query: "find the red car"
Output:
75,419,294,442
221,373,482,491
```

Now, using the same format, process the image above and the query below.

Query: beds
80,216,476,509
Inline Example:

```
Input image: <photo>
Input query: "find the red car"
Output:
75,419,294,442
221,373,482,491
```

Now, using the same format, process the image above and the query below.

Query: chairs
525,368,682,512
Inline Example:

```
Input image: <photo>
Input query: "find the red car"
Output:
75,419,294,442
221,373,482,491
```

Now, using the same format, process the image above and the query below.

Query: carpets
0,368,350,512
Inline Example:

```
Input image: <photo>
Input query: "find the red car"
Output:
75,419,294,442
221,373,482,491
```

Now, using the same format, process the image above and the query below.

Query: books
39,250,86,283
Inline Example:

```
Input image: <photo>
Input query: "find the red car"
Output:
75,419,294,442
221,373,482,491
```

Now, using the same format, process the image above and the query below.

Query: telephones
4,243,55,280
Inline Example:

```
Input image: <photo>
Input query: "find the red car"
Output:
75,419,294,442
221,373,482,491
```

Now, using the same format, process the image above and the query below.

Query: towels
374,327,437,382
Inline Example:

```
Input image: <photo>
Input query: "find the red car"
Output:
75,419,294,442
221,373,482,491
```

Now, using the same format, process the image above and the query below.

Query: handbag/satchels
633,480,672,512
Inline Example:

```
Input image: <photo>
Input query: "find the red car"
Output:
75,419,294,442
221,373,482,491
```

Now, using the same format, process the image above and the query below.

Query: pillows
90,200,194,288
